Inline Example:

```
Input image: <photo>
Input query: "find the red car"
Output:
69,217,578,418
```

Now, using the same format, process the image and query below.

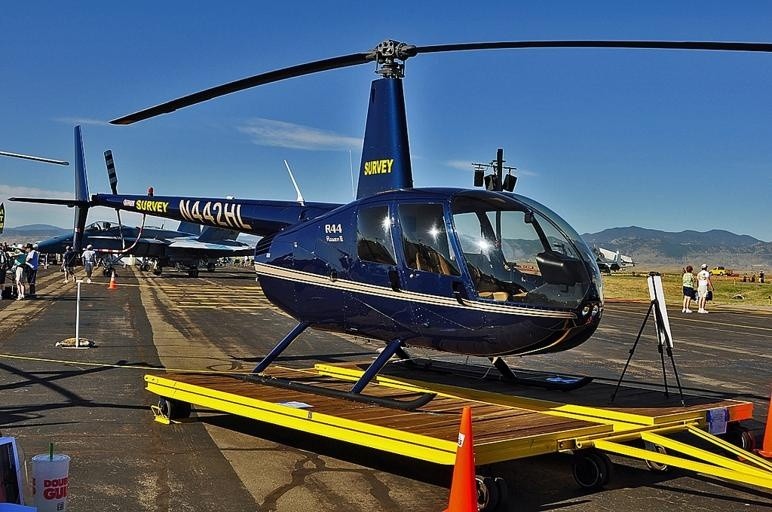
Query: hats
701,264,709,269
25,244,32,248
13,243,25,250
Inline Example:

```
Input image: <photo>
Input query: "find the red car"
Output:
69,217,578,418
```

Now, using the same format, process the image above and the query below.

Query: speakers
503,174,517,192
474,170,484,187
485,174,498,189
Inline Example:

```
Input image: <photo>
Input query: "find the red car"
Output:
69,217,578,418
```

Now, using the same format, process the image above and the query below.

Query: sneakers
682,308,709,314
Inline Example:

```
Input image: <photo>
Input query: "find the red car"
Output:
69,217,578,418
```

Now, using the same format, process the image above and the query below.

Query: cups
32,452,71,512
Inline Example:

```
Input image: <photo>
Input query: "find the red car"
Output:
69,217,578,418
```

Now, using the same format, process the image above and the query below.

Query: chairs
358,240,452,276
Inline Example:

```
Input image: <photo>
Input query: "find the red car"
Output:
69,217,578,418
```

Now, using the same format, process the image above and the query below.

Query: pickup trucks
709,267,734,276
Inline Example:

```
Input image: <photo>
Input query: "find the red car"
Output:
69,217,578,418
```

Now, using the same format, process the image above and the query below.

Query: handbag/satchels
706,290,712,300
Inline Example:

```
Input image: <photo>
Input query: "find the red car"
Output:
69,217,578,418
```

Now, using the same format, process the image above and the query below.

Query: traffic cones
440,408,483,511
107,268,117,290
754,389,772,459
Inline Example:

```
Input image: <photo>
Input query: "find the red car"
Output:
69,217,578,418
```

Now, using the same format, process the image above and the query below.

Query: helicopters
10,41,772,411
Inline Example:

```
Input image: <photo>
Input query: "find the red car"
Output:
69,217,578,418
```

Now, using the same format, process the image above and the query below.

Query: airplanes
589,246,634,273
33,220,257,277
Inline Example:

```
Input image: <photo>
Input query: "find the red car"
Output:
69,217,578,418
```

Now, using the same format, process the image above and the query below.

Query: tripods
609,279,691,408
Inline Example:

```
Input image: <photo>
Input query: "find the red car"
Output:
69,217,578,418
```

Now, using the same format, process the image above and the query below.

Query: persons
696,264,714,314
681,266,696,314
0,241,100,301
750,275,755,282
758,271,764,283
218,255,256,269
